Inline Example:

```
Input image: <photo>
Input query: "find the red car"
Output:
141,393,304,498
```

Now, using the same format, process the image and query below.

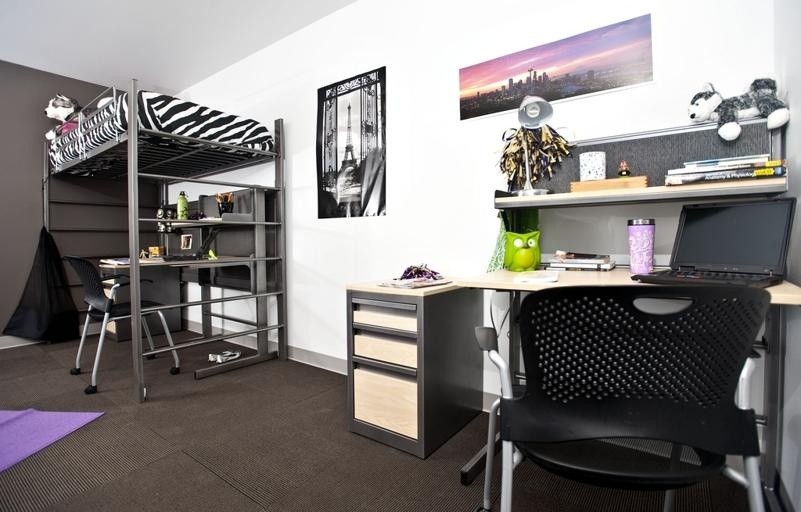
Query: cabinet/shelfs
346,278,484,459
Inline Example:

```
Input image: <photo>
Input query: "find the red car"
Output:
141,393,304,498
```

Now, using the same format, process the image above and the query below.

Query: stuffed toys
43,91,114,140
688,77,790,140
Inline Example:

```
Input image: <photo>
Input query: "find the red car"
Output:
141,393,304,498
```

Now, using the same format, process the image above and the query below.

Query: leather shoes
208,351,241,363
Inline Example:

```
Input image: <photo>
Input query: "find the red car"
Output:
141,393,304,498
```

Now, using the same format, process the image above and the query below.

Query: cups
217,202,235,218
626,217,656,276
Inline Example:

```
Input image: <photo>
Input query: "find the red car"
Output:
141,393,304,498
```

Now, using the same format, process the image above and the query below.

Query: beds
43,80,288,403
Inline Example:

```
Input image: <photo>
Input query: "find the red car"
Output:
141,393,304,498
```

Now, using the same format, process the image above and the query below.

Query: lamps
516,94,553,195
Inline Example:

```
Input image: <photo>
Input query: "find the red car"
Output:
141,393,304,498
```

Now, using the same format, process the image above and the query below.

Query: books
570,175,647,192
537,249,616,272
664,154,787,186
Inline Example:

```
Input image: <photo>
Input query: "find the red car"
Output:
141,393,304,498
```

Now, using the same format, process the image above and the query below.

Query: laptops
630,196,798,288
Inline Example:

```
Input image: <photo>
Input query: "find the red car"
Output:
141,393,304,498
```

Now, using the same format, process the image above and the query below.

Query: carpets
0,408,106,472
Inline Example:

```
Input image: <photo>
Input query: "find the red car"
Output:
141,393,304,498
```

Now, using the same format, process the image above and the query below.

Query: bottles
176,190,189,220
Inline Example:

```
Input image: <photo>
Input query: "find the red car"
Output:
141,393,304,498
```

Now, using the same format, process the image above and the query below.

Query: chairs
62,255,180,394
501,284,771,512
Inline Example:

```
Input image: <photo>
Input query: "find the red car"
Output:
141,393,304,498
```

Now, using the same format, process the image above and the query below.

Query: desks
99,187,279,379
452,178,801,512
